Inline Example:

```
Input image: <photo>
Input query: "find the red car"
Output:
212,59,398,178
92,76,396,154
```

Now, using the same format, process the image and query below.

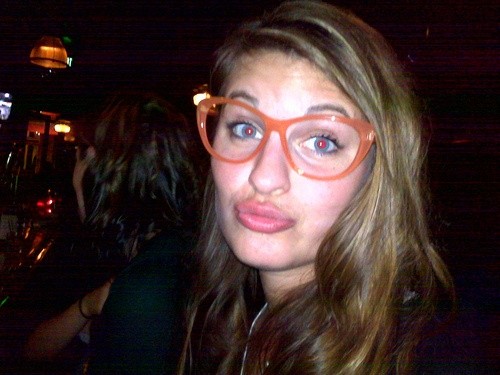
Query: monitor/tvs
0,91,15,122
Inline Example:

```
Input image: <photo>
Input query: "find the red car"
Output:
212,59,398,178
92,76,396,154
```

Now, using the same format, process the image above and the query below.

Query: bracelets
79,296,93,320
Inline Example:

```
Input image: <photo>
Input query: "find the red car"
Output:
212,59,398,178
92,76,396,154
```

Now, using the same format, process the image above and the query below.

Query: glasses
197,99,376,181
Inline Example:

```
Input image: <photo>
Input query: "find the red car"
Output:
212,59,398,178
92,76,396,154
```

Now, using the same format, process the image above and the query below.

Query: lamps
30,36,67,69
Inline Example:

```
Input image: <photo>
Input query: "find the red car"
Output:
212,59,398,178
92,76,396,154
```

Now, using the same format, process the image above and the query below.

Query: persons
71,93,201,375
0,218,117,363
176,0,485,375
